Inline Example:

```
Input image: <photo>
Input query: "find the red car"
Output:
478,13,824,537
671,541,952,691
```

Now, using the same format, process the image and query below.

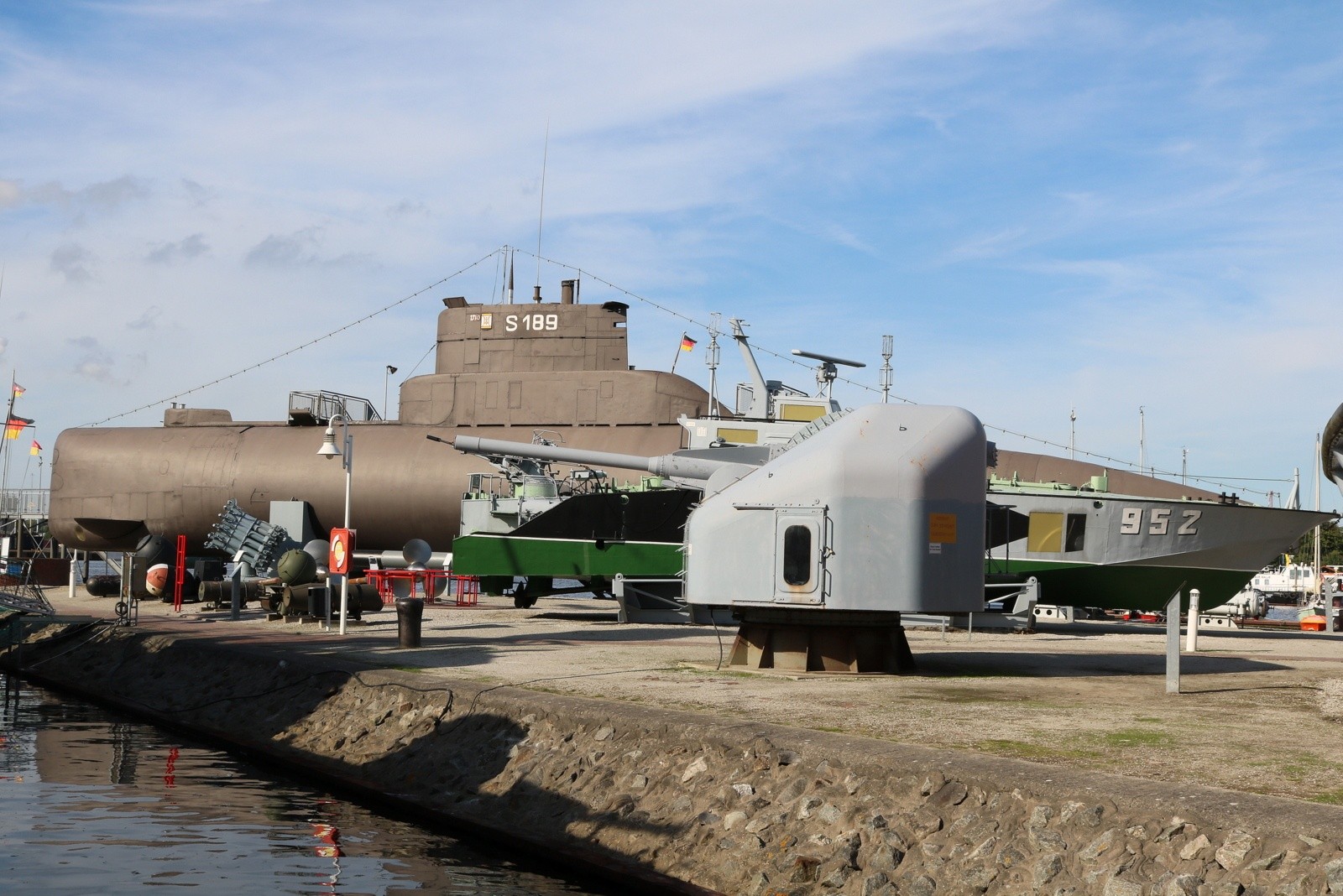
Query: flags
4,414,35,440
681,335,698,352
29,441,43,457
13,382,26,397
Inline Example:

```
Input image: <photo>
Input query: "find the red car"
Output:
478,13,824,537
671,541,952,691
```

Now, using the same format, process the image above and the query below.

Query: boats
1104,552,1343,626
441,310,1343,632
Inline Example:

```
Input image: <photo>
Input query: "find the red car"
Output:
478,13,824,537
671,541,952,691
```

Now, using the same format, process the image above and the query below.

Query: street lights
316,414,353,635
